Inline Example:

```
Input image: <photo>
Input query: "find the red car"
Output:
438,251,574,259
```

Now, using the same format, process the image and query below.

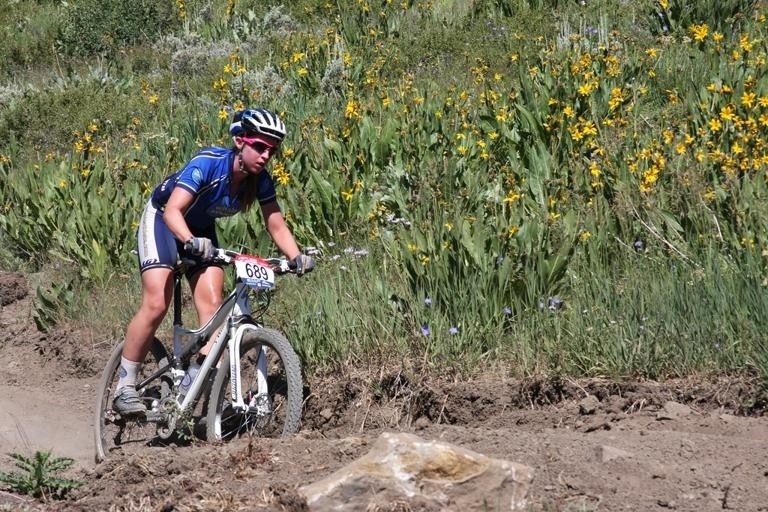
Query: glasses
239,134,278,156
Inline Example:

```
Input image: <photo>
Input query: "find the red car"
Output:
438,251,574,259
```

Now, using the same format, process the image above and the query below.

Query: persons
111,106,318,438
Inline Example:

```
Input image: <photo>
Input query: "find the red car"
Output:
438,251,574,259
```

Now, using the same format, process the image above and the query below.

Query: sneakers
110,386,147,418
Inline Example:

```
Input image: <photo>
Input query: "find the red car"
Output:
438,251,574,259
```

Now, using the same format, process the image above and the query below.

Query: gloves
288,253,317,279
181,236,217,266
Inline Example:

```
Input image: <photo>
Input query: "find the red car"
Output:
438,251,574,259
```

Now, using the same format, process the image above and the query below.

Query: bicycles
89,240,319,464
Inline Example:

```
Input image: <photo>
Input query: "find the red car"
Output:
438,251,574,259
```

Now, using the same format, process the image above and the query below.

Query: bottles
178,355,207,397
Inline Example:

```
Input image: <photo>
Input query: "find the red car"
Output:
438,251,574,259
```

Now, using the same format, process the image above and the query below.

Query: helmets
226,106,288,145
